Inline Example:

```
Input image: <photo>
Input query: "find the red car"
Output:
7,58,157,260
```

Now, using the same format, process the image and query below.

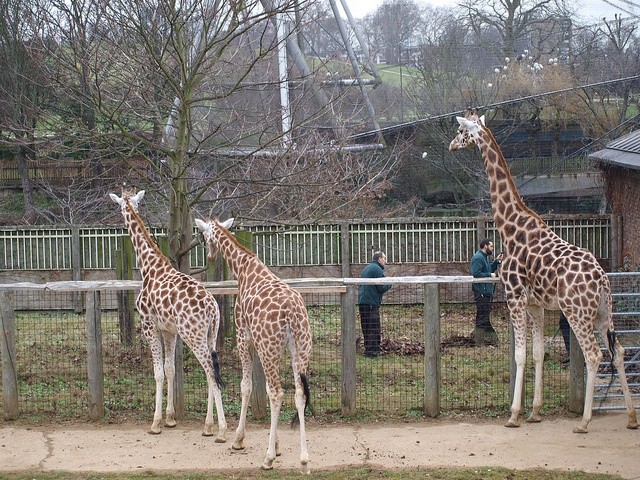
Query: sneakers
562,357,570,364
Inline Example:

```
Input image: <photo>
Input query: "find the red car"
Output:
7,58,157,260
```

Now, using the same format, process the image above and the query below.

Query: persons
357,250,392,357
559,312,571,364
470,238,504,342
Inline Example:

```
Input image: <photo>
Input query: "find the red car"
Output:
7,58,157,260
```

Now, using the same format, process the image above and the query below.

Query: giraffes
108,182,227,443
192,216,316,475
447,108,640,433
